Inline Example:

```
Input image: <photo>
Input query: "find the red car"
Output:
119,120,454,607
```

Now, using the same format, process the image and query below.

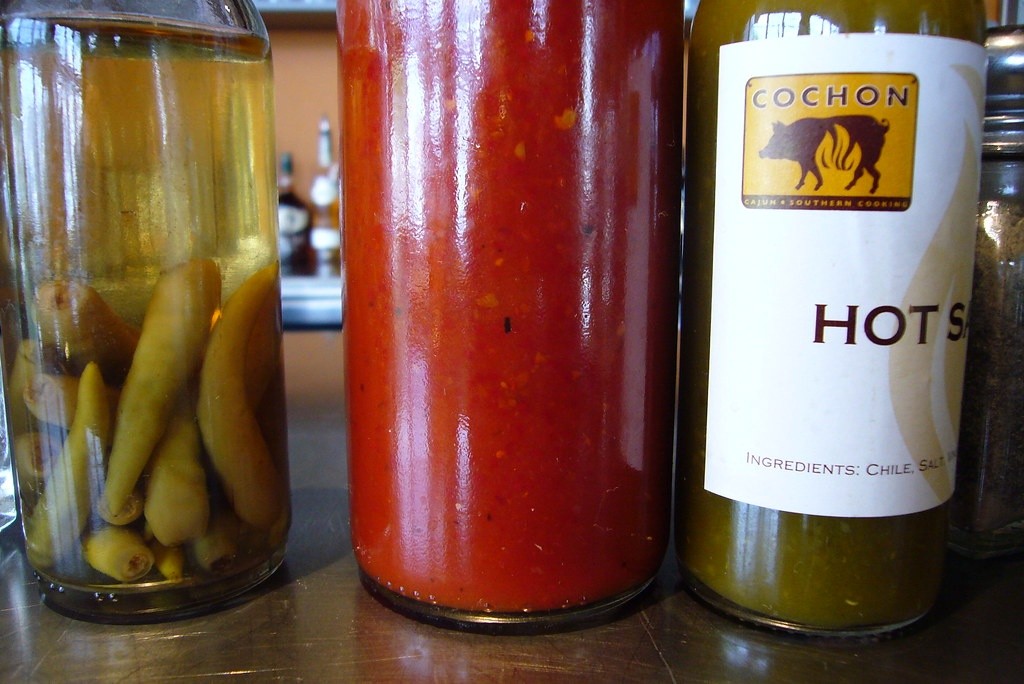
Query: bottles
337,0,691,635
947,32,1024,559
0,0,294,624
307,118,340,274
684,0,986,638
277,154,313,276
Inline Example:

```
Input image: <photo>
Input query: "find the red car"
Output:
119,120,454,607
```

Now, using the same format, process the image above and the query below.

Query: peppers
10,258,292,586
